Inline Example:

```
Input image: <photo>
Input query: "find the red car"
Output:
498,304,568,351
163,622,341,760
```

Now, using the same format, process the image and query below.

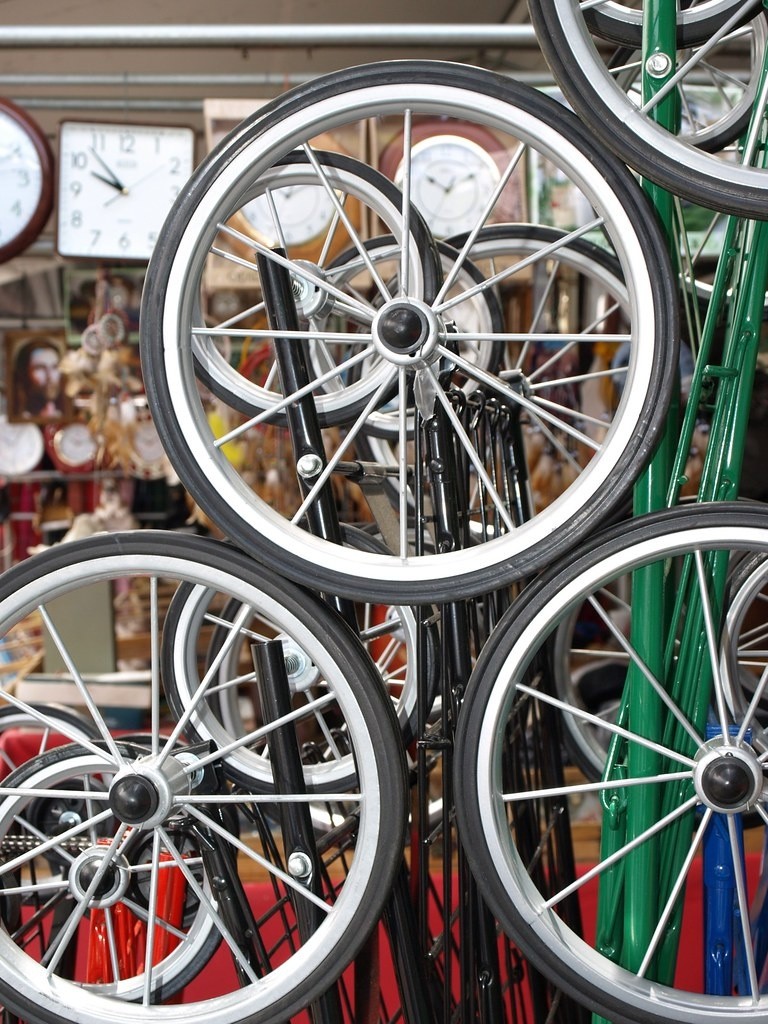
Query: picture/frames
2,326,73,426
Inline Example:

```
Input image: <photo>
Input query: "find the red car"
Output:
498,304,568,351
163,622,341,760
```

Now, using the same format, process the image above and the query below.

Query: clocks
380,121,525,264
0,93,55,262
55,118,197,267
204,97,372,298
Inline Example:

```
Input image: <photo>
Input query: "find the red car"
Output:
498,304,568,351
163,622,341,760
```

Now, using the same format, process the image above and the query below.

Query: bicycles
1,2,768,1024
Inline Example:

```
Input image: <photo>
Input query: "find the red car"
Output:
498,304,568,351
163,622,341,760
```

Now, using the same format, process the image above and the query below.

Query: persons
6,335,73,422
